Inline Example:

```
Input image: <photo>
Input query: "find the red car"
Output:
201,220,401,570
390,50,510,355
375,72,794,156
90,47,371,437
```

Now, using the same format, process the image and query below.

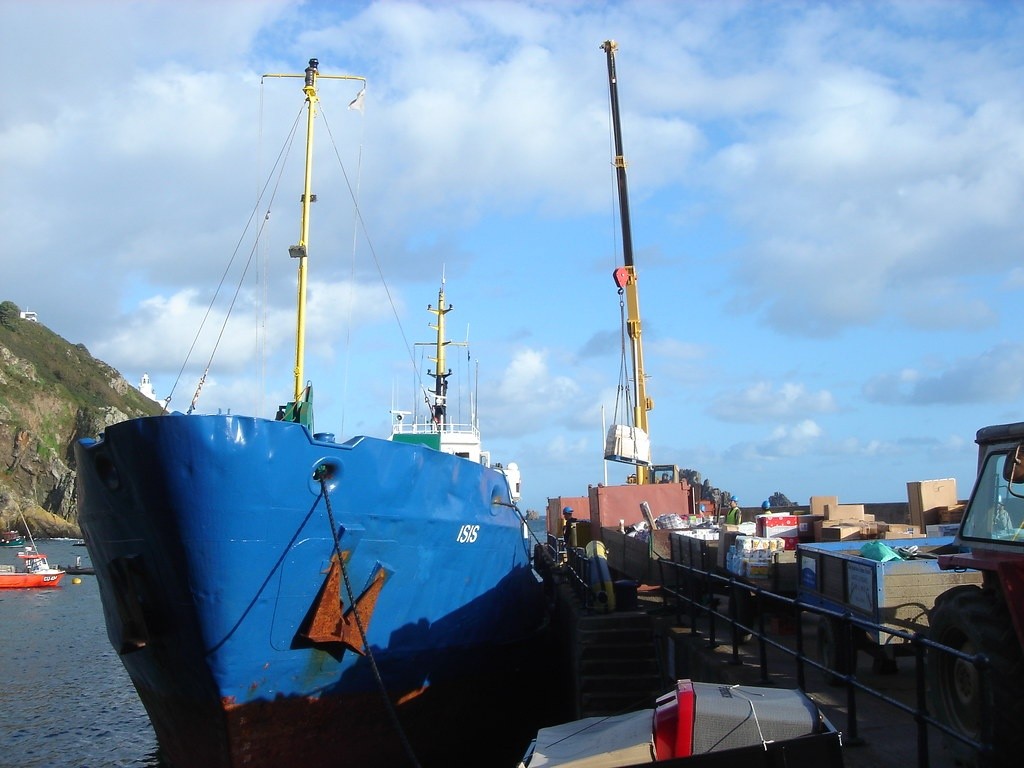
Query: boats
0,536,24,548
72,58,560,767
0,504,66,588
73,541,87,547
59,564,96,576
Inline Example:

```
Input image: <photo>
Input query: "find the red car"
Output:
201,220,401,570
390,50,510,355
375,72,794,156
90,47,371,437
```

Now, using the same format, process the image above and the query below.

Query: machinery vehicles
792,422,1024,768
597,38,680,484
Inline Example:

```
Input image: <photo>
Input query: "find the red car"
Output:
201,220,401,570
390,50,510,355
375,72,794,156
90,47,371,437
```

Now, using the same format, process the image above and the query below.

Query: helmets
762,501,770,509
700,505,704,512
729,495,739,502
563,507,574,514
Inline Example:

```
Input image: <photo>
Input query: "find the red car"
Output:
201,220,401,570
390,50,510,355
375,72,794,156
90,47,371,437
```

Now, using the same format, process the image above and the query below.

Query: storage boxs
716,478,966,579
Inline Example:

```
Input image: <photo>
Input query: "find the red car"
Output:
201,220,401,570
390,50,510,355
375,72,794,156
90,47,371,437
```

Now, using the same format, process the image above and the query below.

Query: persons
25,558,31,567
658,474,669,484
726,495,742,524
762,500,772,514
562,507,579,543
